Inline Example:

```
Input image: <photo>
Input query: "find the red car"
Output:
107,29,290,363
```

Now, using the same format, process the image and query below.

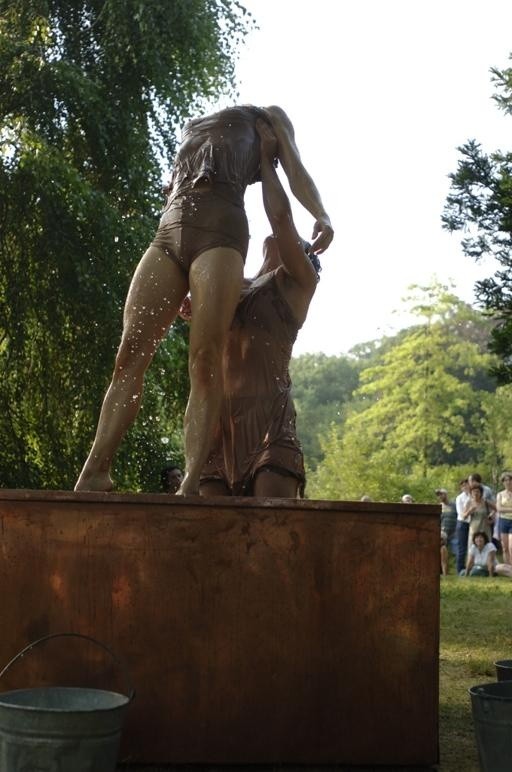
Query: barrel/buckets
493,659,512,681
0,630,137,772
468,680,512,772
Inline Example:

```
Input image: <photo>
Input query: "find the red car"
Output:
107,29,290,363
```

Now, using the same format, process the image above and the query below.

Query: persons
182,116,322,499
76,104,332,496
403,473,512,576
159,467,186,495
